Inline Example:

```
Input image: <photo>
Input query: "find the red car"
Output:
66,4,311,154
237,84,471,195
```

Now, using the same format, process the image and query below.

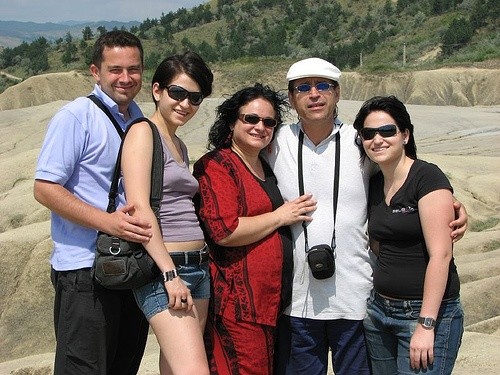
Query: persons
353,95,465,375
32,30,150,375
121,50,215,375
193,82,318,375
258,57,467,375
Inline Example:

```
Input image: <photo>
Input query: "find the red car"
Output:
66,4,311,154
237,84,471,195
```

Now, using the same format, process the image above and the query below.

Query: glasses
236,113,280,128
292,83,337,93
361,124,401,140
162,84,205,106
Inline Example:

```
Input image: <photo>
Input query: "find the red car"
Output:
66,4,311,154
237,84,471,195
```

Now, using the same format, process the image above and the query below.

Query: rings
181,298,187,303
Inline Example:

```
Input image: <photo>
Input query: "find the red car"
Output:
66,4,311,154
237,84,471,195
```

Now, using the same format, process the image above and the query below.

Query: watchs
418,317,436,328
162,270,178,282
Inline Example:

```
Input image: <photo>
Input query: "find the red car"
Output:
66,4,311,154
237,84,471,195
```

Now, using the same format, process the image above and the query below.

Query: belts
170,248,209,265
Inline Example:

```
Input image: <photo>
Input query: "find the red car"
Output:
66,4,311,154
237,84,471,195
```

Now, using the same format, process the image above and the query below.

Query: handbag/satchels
308,244,336,280
89,233,161,289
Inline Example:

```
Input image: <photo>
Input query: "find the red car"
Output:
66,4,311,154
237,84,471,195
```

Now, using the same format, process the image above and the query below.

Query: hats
286,57,342,81
